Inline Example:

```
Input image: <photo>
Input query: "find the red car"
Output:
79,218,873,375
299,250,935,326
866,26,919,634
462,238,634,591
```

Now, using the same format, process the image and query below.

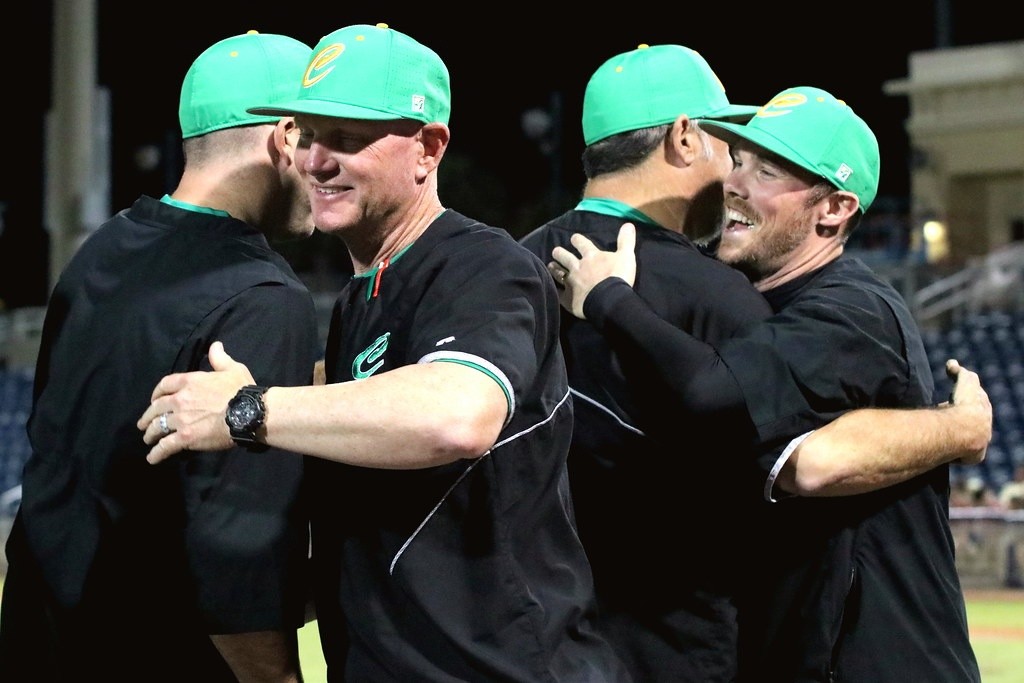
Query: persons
137,22,629,682
548,85,982,679
0,29,316,683
516,46,993,683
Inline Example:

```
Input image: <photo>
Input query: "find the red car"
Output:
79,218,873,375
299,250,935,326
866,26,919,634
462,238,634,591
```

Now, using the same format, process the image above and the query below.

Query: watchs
225,383,275,455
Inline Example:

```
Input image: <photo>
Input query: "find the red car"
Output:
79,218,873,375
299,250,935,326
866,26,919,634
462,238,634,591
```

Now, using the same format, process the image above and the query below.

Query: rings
558,271,569,285
159,413,170,434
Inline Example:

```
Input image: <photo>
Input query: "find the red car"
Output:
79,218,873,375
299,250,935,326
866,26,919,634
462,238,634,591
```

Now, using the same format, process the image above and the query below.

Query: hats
582,44,764,146
245,23,451,127
698,87,880,215
179,30,313,138
